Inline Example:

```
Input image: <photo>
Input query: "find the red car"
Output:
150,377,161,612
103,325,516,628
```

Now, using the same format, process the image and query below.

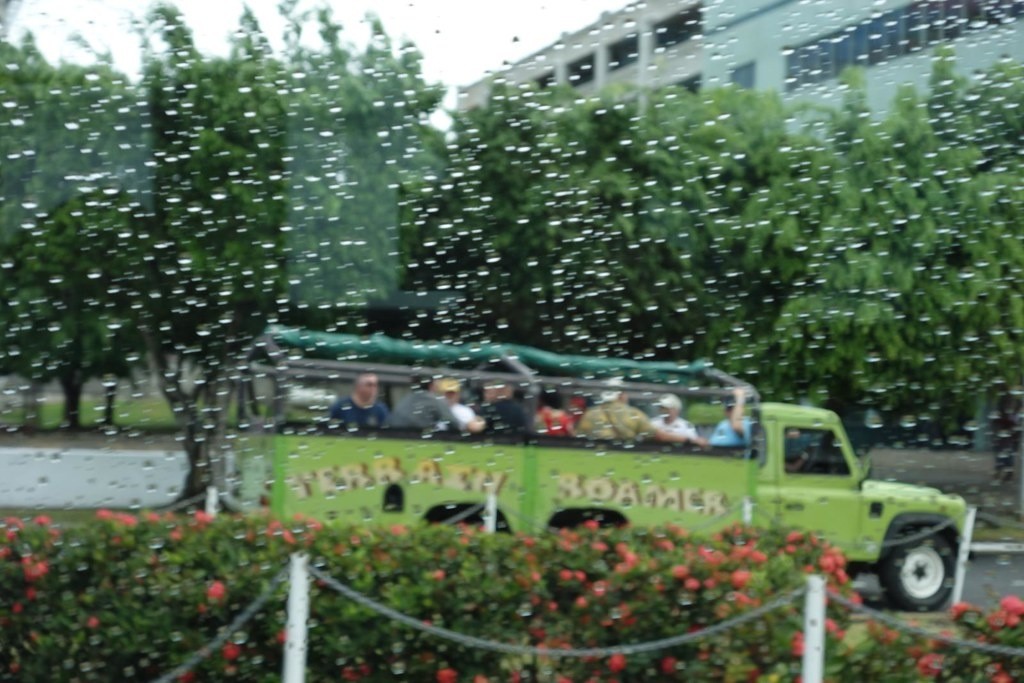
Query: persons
327,371,813,472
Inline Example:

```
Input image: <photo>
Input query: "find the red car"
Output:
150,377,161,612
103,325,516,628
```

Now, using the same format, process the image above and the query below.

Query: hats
651,393,685,411
724,396,737,407
440,378,460,394
600,379,623,400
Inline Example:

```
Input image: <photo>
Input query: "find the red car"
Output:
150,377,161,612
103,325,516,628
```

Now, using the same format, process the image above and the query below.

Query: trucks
220,336,978,613
